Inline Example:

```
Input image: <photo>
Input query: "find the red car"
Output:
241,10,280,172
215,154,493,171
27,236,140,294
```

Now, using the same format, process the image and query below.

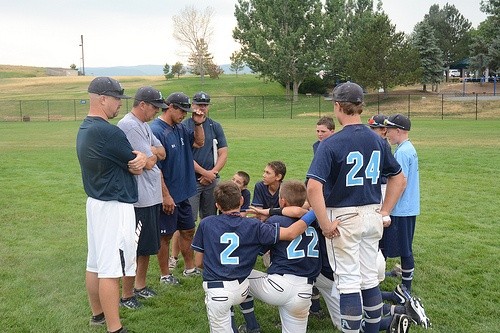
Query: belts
277,273,314,284
207,278,246,289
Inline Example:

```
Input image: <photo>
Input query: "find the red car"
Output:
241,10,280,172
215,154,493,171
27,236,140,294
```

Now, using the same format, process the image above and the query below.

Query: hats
135,86,168,109
193,92,210,104
88,76,131,99
166,92,191,112
381,114,411,131
332,81,364,102
365,114,388,128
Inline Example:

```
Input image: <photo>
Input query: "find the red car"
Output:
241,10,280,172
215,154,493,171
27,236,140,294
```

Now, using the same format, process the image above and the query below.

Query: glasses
383,119,404,128
367,118,384,126
101,89,124,95
152,97,165,103
193,98,210,102
175,102,191,108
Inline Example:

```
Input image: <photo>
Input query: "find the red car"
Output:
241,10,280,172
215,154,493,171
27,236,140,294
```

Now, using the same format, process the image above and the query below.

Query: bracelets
193,121,202,126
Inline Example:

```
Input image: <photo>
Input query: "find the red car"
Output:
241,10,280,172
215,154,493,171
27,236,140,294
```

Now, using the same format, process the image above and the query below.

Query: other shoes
120,329,135,333
90,318,106,327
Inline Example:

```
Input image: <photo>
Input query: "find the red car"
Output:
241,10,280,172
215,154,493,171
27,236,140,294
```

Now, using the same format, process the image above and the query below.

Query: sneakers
169,256,178,270
309,308,324,319
392,263,413,279
238,325,261,333
133,287,157,299
389,314,411,333
183,268,203,277
394,284,412,304
406,294,432,330
160,274,178,285
120,296,143,310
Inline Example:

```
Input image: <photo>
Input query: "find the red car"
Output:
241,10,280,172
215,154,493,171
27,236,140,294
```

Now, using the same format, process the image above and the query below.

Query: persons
115,86,167,311
304,81,407,333
150,91,205,278
378,115,421,300
227,113,433,333
74,77,148,333
190,181,317,333
182,91,228,221
